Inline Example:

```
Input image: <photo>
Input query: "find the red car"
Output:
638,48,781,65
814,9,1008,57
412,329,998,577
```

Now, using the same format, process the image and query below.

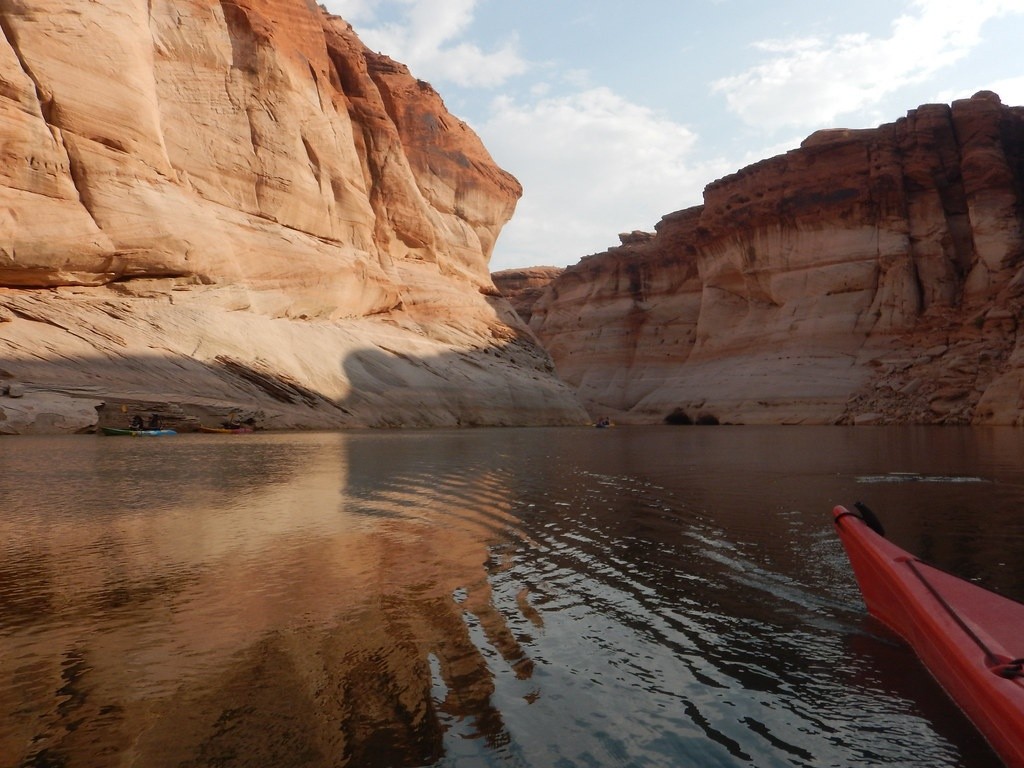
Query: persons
129,415,144,431
148,414,161,430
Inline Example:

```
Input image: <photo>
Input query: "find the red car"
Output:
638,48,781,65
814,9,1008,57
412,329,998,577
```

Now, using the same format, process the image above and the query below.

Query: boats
585,422,615,428
192,423,254,434
101,426,176,436
830,505,1024,768
